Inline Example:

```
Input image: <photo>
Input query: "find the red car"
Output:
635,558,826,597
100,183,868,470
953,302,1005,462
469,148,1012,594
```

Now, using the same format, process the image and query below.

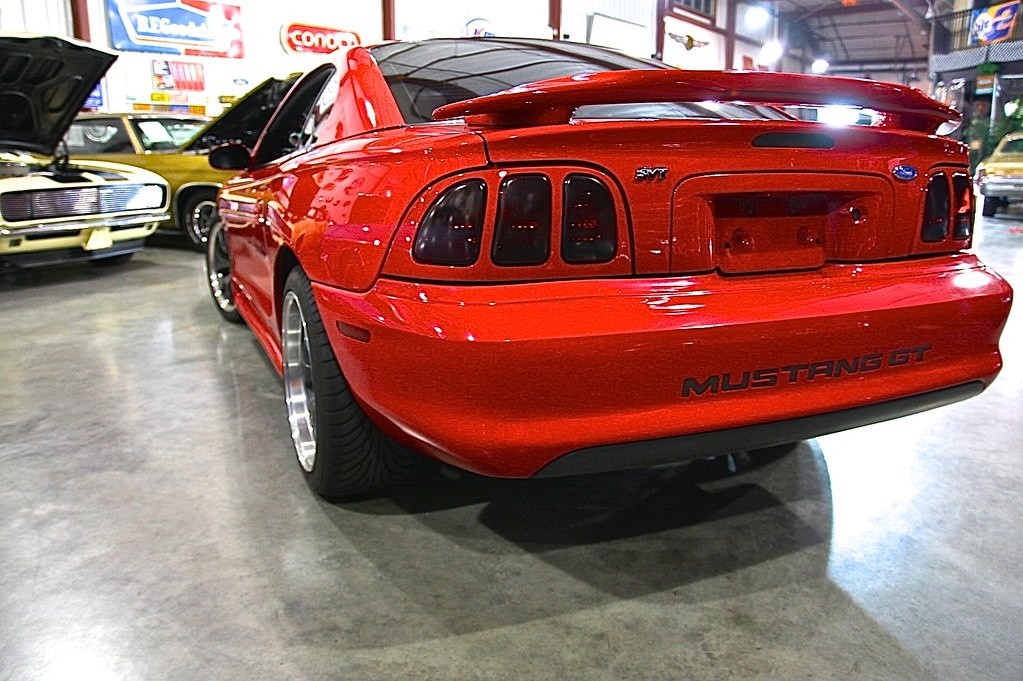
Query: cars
48,68,305,253
1,33,173,270
973,130,1023,219
201,35,1011,500
